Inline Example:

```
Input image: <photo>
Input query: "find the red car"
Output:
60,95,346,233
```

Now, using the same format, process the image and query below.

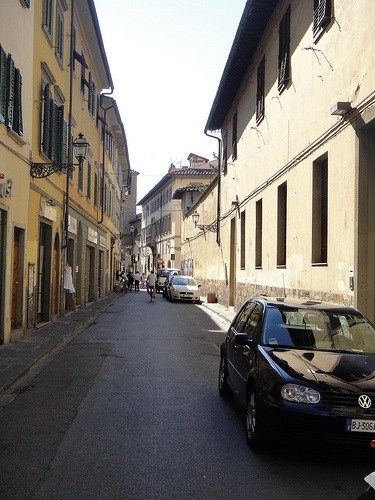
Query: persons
146,270,157,300
140,273,146,289
118,271,129,292
133,271,140,292
126,271,134,292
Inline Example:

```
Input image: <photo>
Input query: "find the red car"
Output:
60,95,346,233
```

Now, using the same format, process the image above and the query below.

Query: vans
157,268,181,293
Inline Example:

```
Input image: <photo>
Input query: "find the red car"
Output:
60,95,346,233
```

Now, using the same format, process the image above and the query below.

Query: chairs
302,312,323,341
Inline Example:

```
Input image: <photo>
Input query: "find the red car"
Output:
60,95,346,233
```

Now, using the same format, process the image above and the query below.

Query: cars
162,275,201,303
218,297,375,451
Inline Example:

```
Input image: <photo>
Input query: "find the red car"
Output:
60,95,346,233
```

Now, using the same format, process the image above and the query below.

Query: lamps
331,101,352,116
166,240,180,251
46,200,56,207
192,210,217,235
30,132,91,180
